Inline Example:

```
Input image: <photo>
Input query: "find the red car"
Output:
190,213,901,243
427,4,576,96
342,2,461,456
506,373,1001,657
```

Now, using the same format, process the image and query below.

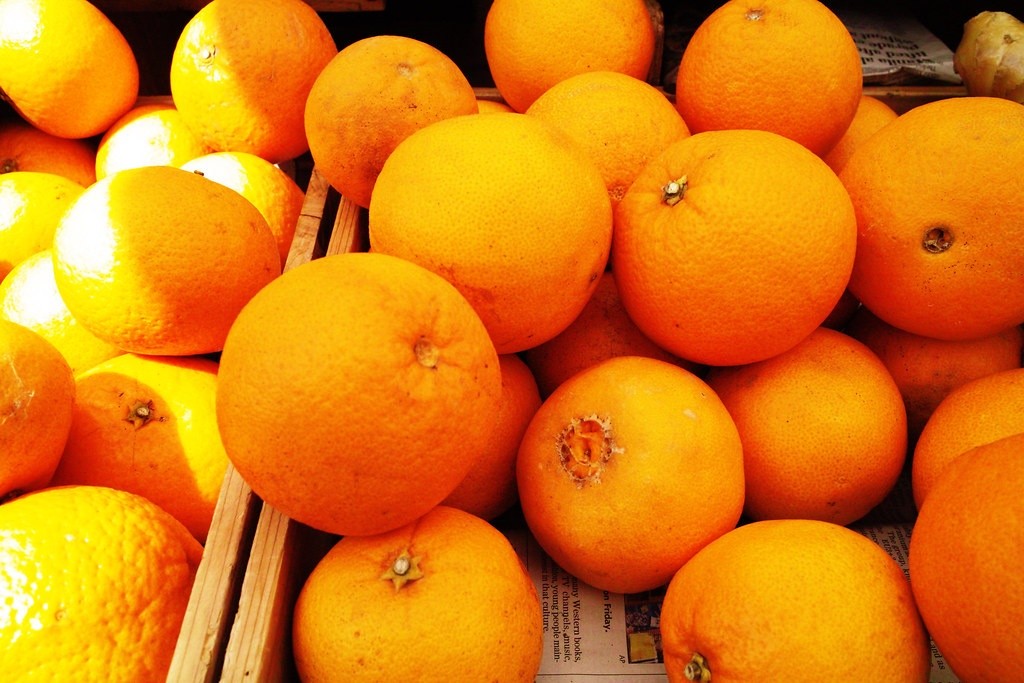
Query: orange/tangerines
0,0,1024,683
